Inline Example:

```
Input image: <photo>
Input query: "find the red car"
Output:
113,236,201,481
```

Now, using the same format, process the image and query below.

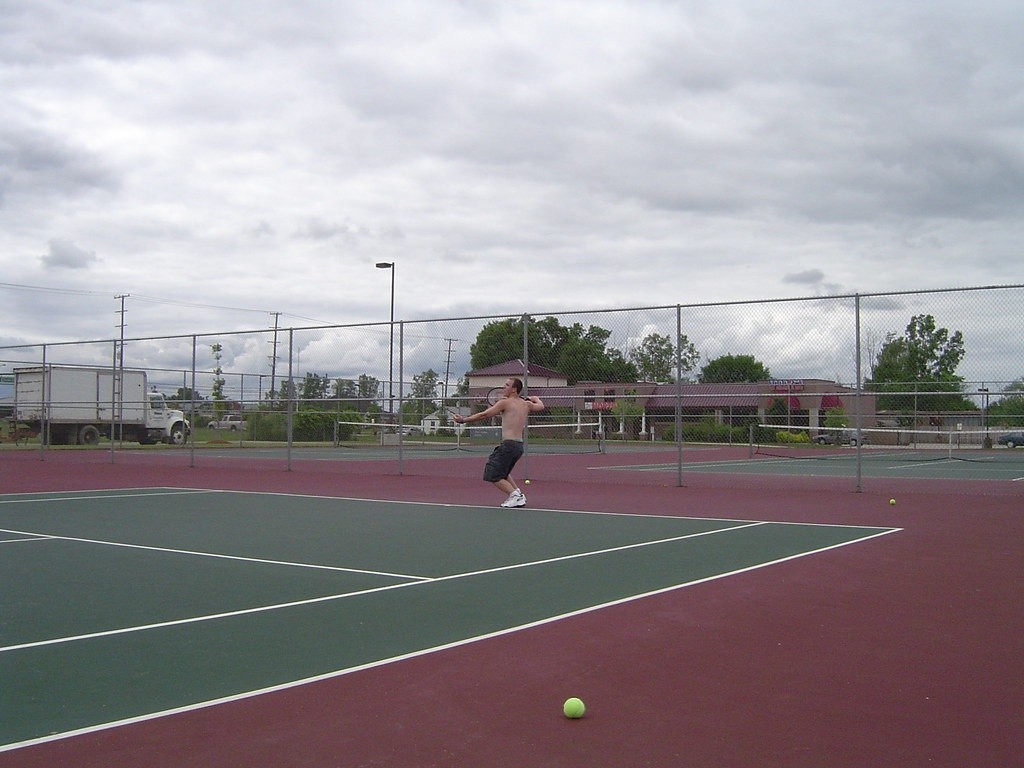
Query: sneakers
501,494,527,508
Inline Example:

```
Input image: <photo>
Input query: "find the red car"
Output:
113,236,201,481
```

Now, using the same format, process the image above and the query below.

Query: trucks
13,366,191,446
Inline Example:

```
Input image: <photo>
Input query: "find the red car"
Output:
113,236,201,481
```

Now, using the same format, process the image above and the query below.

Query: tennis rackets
487,387,530,407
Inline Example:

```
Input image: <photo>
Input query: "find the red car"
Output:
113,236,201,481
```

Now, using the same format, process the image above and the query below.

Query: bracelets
461,417,465,423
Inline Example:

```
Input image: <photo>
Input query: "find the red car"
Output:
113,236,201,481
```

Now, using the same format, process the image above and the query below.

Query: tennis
524,480,530,485
889,498,896,505
562,696,586,719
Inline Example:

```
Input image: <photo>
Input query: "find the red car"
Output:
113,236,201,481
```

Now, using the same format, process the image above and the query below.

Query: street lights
376,262,393,435
978,388,993,438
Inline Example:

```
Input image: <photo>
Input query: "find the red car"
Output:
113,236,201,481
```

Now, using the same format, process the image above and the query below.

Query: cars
998,432,1024,449
397,426,426,437
207,414,248,432
812,429,867,446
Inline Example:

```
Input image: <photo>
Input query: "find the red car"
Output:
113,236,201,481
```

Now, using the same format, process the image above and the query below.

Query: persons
452,377,544,508
